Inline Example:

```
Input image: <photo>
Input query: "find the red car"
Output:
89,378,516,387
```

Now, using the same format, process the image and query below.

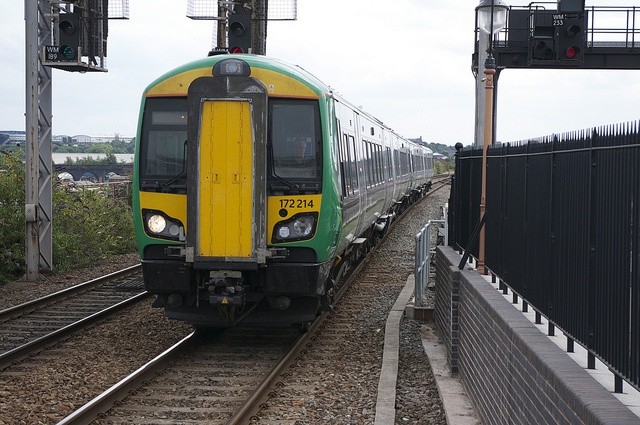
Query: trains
132,55,433,327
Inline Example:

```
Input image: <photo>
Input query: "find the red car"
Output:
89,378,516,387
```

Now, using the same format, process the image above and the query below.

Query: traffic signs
59,11,84,63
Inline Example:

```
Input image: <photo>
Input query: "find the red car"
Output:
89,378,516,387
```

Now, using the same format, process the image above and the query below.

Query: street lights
478,1,509,274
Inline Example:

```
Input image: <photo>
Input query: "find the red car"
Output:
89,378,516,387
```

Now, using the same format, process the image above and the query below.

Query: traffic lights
555,11,589,60
228,3,253,54
